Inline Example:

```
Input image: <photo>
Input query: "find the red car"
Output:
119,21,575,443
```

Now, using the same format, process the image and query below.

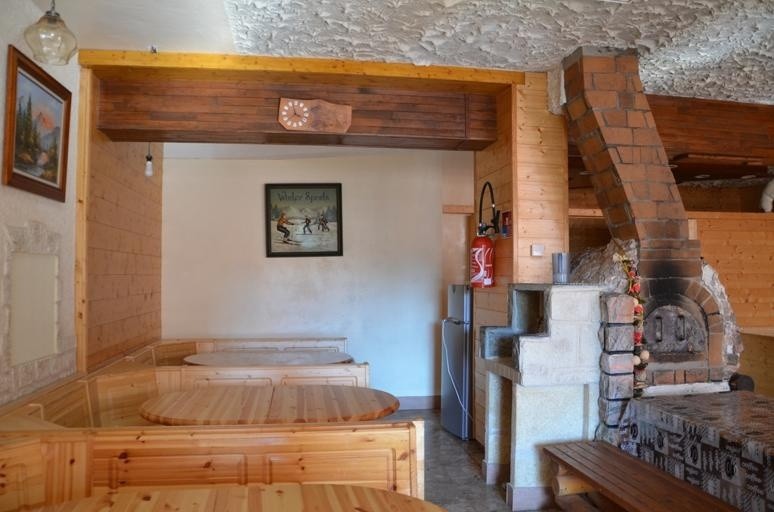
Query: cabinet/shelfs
480,281,618,511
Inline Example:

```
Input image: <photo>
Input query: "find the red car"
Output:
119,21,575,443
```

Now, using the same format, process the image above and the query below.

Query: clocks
278,96,354,136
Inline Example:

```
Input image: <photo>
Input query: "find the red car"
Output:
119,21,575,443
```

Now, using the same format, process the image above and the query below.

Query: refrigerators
440,283,472,439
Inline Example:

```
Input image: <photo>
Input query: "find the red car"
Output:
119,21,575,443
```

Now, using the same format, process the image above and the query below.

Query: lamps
21,0,80,67
144,140,154,178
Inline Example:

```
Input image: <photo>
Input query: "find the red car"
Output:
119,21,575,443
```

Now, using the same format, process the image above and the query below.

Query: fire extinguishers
469,181,500,288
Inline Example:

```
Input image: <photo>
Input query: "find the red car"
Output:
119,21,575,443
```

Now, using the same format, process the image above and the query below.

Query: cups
550,253,570,284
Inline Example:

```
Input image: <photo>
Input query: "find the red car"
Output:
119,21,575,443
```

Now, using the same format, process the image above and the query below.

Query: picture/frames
2,40,73,205
263,181,344,259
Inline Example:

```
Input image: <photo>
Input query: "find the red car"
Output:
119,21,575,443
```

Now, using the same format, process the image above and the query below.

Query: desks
623,384,774,512
135,385,402,427
182,351,356,367
21,481,447,512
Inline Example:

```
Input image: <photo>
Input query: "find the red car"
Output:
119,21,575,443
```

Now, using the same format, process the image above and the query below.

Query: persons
276,213,330,243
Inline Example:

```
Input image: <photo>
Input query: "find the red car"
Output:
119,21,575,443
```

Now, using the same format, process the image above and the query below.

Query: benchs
536,438,744,510
